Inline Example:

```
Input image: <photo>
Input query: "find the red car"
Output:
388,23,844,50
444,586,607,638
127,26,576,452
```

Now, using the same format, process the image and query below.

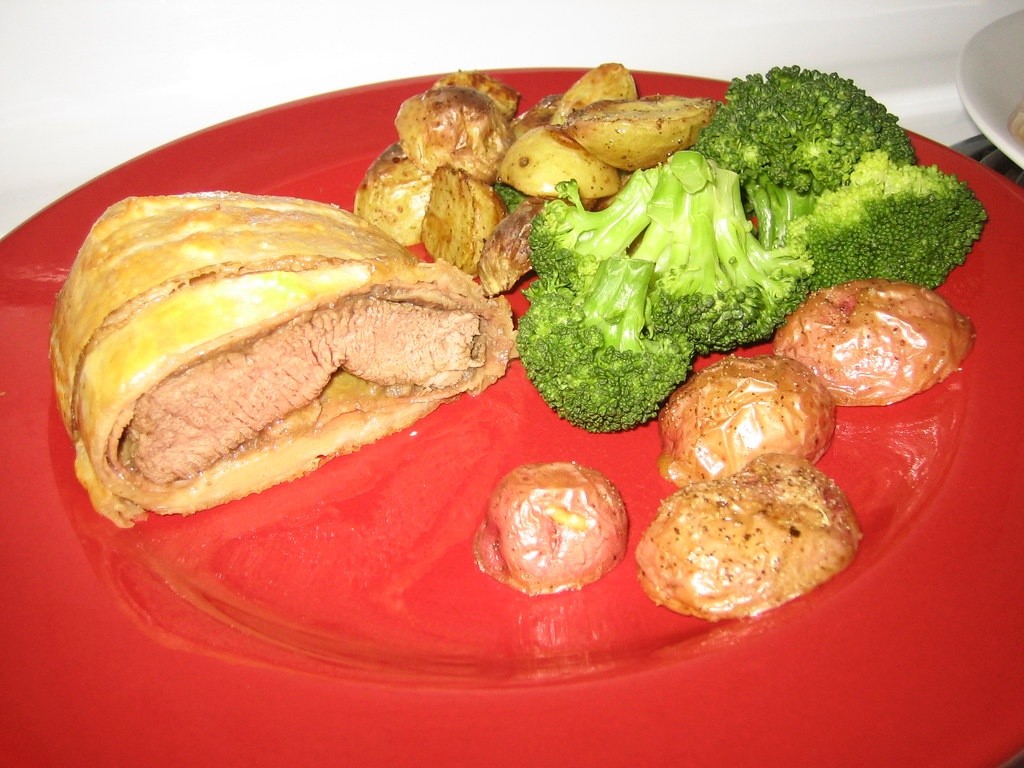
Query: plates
954,8,1024,171
0,66,1024,768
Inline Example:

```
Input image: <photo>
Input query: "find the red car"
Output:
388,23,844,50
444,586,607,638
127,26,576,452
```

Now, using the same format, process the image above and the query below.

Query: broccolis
496,64,987,434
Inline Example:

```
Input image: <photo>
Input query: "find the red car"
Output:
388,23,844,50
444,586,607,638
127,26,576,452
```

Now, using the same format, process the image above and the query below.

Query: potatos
471,281,974,622
349,63,718,274
